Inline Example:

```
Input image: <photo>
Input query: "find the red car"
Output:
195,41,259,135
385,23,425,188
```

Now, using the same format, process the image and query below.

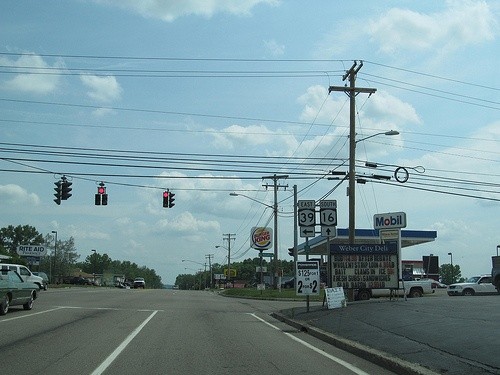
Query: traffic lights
62,182,72,200
95,187,108,205
54,182,62,205
163,192,168,207
169,192,175,208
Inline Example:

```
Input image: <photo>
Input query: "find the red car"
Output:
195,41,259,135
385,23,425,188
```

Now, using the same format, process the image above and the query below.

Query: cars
447,274,497,296
0,264,48,315
283,277,294,289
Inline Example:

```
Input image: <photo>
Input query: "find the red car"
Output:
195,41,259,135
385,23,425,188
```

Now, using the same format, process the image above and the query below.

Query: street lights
448,252,453,284
497,245,500,256
182,260,207,291
349,130,399,244
215,245,230,283
230,192,278,268
52,231,57,284
91,250,96,286
185,268,202,291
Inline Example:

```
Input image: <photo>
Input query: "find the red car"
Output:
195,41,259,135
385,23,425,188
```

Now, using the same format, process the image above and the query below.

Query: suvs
134,278,145,289
72,277,91,286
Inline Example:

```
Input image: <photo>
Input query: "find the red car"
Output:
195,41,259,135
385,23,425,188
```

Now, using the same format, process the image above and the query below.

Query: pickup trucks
355,281,432,300
